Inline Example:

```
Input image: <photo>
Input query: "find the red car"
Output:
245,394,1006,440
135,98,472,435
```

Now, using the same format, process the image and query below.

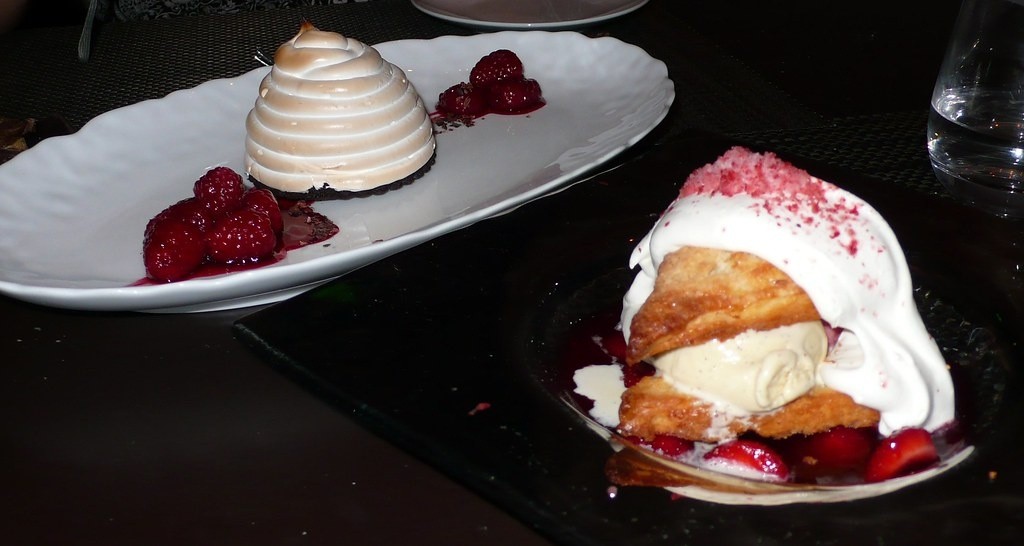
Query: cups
926,0,1024,223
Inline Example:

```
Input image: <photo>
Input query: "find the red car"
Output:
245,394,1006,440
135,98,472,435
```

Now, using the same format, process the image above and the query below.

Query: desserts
568,146,960,505
241,21,436,202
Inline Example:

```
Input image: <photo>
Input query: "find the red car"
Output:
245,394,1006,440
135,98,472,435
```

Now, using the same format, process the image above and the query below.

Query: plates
536,266,1007,506
0,29,677,314
410,0,650,29
